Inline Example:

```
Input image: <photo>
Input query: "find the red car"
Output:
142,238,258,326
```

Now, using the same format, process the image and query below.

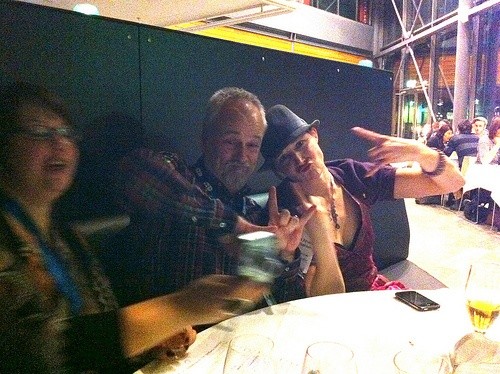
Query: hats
256,105,321,172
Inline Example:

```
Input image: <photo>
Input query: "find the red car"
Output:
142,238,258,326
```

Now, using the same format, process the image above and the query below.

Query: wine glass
464,264,500,335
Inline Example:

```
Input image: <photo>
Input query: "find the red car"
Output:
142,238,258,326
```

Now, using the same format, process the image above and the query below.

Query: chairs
458,156,479,213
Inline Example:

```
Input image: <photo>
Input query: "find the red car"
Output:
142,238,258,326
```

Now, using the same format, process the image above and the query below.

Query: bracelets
420,146,446,177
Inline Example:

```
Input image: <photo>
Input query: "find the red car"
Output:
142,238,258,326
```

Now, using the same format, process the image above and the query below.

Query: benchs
74,192,447,331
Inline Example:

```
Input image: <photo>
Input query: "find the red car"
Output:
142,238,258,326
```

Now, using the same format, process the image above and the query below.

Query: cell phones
395,291,440,311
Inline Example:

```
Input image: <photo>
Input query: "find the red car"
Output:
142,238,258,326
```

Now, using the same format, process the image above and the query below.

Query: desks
135,288,500,374
463,162,500,231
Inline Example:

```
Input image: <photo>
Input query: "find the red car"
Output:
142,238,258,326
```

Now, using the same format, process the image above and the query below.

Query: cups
299,341,357,374
393,350,442,374
223,334,274,374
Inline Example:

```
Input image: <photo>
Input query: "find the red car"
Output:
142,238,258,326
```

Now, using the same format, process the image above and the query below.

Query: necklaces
299,176,341,229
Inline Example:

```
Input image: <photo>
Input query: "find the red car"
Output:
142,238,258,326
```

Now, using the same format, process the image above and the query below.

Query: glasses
12,125,83,143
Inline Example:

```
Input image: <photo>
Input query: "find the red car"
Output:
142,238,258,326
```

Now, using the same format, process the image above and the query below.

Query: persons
258,104,467,299
107,86,317,332
415,116,500,224
0,79,268,374
